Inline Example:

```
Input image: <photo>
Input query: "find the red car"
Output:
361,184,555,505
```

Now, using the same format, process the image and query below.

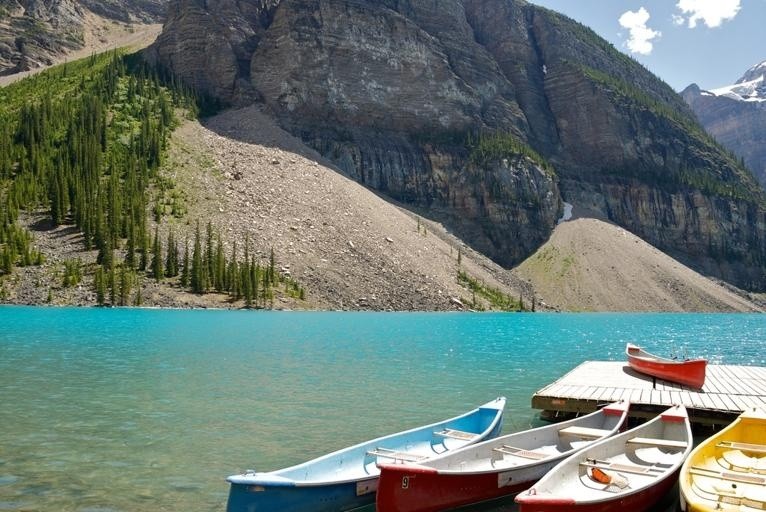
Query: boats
366,395,630,511
626,342,707,390
513,402,694,511
679,404,766,511
225,395,508,511
